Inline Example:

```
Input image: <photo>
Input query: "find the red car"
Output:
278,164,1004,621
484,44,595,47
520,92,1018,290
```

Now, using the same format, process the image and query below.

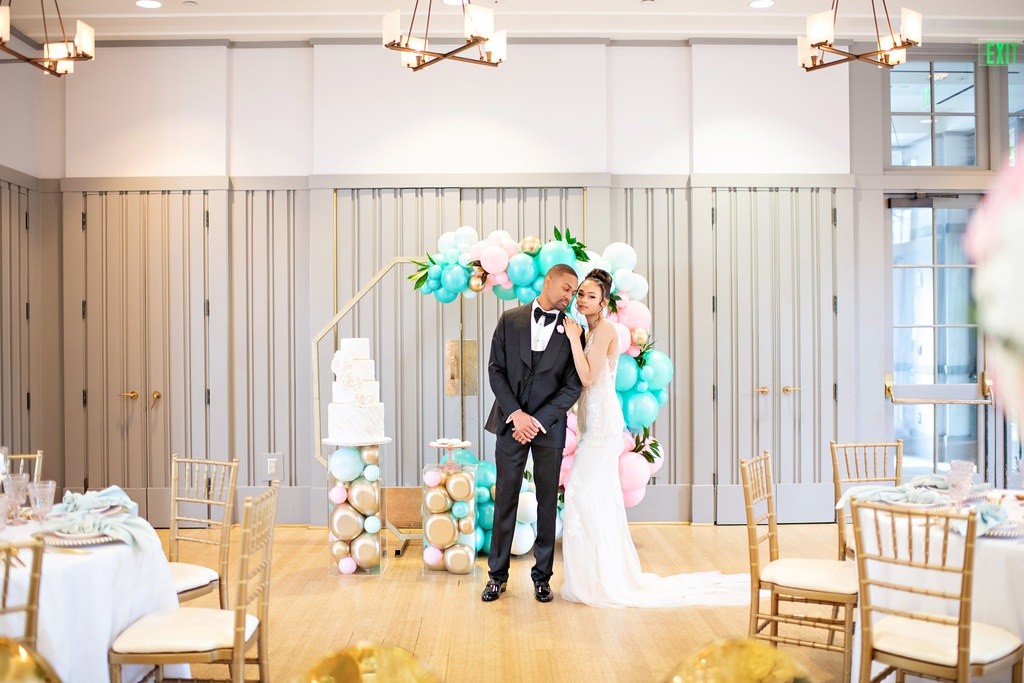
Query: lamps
382,0,508,71
797,0,923,72
0,0,96,77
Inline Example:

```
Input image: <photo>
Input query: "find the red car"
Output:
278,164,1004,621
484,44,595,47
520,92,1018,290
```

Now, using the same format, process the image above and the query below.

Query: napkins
42,485,138,547
835,473,1007,538
737,449,858,683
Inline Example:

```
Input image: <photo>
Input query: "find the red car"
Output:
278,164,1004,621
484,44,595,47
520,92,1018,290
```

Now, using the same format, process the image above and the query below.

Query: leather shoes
481,579,507,601
534,581,554,602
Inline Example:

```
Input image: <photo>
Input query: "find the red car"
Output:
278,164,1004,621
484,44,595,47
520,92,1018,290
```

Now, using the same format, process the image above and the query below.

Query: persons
481,264,586,602
561,268,642,607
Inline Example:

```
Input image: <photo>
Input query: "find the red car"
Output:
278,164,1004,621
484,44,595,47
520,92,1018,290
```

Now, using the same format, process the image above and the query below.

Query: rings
525,432,528,435
566,321,569,323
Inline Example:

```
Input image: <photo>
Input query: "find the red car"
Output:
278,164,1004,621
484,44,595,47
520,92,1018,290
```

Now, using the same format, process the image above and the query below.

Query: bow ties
534,307,556,327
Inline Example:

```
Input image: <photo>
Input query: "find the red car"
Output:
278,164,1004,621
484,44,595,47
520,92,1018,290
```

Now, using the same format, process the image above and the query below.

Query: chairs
849,494,1024,683
5,450,43,496
0,532,47,656
168,455,240,680
826,438,904,646
107,481,279,683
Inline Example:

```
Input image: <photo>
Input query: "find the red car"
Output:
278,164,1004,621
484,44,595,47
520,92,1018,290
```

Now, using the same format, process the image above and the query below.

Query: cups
951,461,973,472
0,447,8,480
0,494,8,529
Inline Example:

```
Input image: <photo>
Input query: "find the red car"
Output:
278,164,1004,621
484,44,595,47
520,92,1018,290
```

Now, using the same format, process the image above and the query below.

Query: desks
850,488,1024,683
0,505,192,683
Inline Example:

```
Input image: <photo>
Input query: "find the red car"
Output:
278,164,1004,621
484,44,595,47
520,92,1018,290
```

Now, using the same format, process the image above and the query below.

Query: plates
881,498,945,508
90,506,123,517
90,506,110,514
54,530,101,537
29,526,117,547
983,521,1024,537
926,485,949,494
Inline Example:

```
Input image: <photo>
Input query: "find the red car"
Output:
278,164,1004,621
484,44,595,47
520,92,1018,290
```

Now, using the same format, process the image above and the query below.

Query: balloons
419,226,673,575
327,446,382,574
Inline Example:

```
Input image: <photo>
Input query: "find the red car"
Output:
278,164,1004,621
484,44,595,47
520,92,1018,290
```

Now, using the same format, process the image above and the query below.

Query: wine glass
3,473,29,525
949,472,972,507
27,480,57,529
